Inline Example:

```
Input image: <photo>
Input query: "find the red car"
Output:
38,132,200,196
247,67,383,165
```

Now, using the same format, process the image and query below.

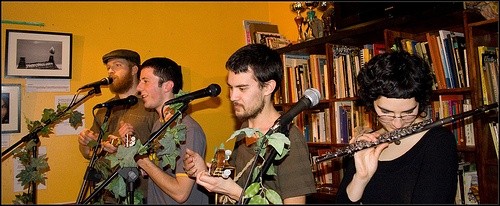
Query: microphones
96,95,139,108
82,77,113,89
265,88,321,137
164,84,221,105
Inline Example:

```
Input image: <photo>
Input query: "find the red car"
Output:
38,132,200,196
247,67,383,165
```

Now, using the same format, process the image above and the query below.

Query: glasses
375,108,420,122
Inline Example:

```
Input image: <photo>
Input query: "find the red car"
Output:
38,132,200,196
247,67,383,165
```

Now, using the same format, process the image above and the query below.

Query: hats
102,49,140,64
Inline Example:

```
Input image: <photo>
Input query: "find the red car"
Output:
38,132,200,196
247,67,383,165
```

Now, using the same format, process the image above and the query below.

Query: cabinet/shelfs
271,0,499,205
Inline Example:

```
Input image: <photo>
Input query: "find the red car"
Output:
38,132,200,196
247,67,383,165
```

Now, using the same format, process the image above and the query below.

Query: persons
76,49,159,205
101,56,208,204
182,43,316,205
333,50,459,206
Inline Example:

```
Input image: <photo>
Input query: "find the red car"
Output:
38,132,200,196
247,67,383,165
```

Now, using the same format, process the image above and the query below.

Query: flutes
312,102,500,166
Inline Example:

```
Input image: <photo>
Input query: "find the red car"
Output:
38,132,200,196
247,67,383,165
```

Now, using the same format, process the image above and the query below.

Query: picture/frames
4,28,72,79
1,84,20,133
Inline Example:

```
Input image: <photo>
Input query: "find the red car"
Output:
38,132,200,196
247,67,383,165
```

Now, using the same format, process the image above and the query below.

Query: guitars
206,148,236,206
87,133,136,160
100,107,183,205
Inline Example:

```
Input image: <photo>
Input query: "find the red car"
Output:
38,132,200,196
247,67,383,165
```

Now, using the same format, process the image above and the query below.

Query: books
308,153,332,192
293,109,332,143
455,161,480,204
283,53,330,102
332,43,385,97
334,101,382,143
426,95,475,146
391,30,470,90
489,123,500,159
478,45,500,105
252,29,288,50
